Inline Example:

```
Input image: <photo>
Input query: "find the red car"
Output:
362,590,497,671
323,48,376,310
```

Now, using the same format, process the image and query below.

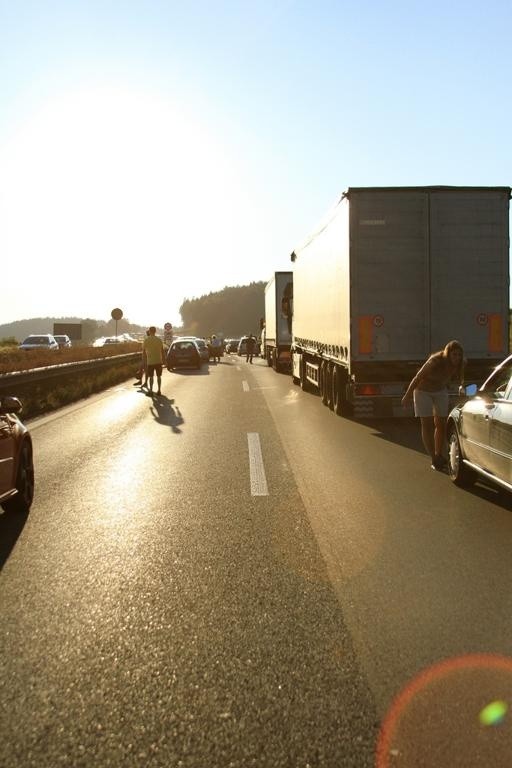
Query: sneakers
146,392,153,395
133,382,142,385
156,391,160,395
431,455,448,472
141,383,148,388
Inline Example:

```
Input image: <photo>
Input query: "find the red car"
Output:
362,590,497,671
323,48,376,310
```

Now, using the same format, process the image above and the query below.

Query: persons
400,339,466,472
211,333,221,364
142,327,165,397
244,332,256,365
132,330,150,389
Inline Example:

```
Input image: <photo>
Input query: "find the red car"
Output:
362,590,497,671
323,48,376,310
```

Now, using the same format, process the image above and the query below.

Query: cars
166,335,209,370
0,394,33,510
17,334,59,350
103,338,120,346
444,355,512,492
223,335,261,357
54,335,72,348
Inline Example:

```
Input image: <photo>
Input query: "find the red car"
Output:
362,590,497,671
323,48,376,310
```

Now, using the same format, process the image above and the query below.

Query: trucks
292,184,512,415
266,272,293,373
